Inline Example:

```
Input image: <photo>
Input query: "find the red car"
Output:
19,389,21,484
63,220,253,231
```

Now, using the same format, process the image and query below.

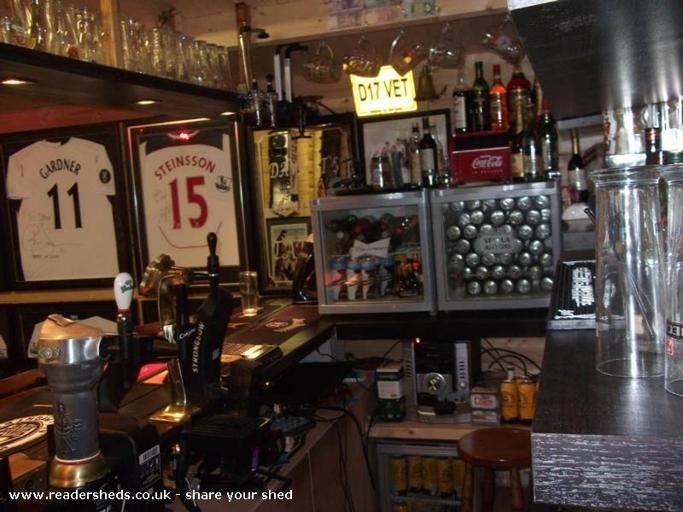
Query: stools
458,426,531,510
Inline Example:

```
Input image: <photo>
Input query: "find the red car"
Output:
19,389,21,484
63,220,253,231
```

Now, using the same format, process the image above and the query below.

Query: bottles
566,138,588,206
640,124,663,163
506,366,515,380
509,89,556,182
449,59,535,134
320,213,418,300
368,115,444,189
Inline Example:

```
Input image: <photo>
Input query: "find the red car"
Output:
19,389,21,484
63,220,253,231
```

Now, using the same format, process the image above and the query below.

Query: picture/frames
0,109,140,291
118,111,252,285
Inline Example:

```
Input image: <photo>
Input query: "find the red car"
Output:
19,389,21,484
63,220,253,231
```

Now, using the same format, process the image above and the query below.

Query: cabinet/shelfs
246,389,376,512
429,180,562,311
310,188,436,315
376,439,486,511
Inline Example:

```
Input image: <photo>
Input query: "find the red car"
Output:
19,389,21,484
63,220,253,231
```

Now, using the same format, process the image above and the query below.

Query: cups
588,167,667,381
336,34,382,77
656,164,682,397
478,24,525,63
239,270,259,317
386,28,430,77
0,0,236,90
422,22,462,69
303,40,341,84
